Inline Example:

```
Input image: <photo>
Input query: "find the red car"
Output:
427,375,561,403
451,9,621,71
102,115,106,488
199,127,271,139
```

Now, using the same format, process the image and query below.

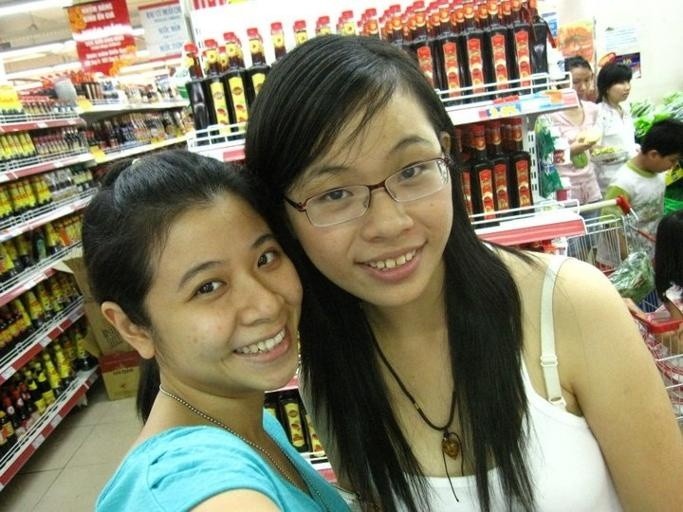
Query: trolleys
561,197,680,421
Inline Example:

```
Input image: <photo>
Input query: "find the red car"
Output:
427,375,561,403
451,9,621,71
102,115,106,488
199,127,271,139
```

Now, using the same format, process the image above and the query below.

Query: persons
594,62,643,199
244,31,683,512
79,146,358,512
599,116,683,263
534,54,604,218
622,206,682,349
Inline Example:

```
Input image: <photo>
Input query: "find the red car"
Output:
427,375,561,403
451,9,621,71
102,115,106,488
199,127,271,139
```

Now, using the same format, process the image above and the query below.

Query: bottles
0,324,97,469
447,116,535,231
0,83,77,124
36,70,184,104
84,109,192,154
0,167,101,231
0,270,82,370
0,206,86,292
182,0,550,148
0,125,91,171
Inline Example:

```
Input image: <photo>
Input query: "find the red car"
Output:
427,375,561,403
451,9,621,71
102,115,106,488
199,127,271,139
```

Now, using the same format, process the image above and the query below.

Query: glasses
284,147,451,229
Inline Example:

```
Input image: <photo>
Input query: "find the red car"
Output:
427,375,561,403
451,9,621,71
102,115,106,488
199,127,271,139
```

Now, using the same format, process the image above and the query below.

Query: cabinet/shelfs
0,70,587,493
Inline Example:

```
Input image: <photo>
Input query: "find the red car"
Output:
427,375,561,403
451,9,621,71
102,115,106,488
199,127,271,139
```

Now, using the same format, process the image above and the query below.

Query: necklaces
157,382,330,512
364,308,468,462
